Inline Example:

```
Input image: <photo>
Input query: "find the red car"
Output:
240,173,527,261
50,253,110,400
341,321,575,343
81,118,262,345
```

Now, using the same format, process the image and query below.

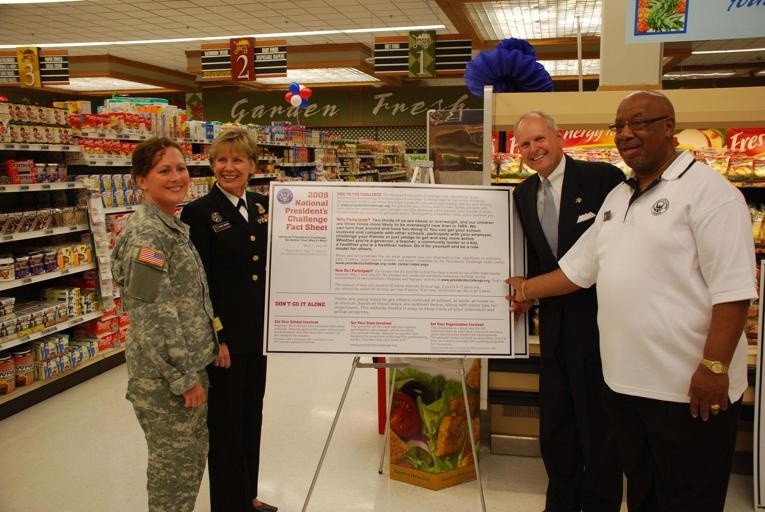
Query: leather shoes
254,501,277,512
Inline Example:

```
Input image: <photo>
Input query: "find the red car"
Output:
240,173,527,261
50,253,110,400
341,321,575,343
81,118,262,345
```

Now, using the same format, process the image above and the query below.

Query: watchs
700,358,729,374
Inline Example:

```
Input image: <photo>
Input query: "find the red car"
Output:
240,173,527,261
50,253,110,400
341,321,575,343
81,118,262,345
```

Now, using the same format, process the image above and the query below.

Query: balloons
285,82,313,108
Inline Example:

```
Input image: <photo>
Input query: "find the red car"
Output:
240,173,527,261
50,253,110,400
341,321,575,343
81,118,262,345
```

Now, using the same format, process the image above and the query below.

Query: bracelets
521,280,527,301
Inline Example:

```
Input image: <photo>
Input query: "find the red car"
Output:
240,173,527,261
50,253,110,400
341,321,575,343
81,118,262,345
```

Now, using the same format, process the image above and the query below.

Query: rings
711,404,721,410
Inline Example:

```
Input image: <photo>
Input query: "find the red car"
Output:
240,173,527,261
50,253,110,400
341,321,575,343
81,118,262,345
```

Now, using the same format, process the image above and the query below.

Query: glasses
608,114,667,133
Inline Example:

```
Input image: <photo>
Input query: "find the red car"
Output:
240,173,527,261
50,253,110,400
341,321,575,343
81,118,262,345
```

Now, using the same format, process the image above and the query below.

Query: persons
180,129,283,512
513,111,626,512
110,137,219,512
504,90,759,512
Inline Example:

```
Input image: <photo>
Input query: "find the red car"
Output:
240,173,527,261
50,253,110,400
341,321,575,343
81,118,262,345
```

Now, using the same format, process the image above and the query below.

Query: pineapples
637,1,686,34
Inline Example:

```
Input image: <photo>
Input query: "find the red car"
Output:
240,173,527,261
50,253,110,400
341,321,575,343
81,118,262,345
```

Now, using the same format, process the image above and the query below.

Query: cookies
435,358,480,460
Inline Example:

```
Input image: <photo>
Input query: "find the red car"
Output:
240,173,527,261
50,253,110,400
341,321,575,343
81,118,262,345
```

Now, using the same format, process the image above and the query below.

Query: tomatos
390,391,422,439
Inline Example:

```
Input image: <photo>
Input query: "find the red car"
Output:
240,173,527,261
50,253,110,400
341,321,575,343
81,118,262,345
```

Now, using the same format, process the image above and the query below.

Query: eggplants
399,380,442,405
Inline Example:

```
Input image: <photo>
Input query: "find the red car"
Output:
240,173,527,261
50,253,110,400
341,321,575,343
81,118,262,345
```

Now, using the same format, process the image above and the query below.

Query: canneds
1,348,35,394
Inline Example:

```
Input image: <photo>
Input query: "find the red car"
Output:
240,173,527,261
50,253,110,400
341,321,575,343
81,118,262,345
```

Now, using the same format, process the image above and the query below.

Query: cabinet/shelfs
474,83,765,462
0,84,424,418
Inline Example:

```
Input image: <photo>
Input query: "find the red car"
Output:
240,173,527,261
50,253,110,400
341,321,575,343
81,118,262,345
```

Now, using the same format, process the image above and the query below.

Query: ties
540,179,558,259
236,198,248,221
